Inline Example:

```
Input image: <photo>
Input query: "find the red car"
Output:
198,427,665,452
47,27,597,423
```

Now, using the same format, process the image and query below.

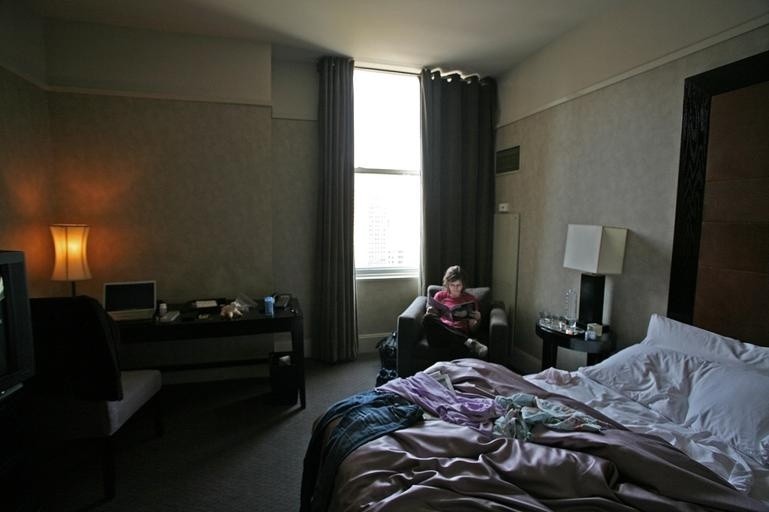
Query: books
428,295,476,322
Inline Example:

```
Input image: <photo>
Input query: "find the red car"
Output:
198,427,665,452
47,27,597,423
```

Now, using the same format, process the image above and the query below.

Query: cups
265,300,273,316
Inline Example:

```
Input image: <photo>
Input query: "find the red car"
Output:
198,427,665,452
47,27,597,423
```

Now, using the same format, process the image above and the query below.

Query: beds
298,312,769,512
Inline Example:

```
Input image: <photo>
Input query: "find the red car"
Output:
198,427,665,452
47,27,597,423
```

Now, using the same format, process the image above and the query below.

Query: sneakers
471,341,488,358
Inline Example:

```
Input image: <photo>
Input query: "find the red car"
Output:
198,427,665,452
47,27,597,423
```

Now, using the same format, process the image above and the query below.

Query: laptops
103,280,156,321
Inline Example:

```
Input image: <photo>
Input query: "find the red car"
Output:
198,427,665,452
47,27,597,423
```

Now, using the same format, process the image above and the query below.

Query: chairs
395,284,511,378
28,296,165,502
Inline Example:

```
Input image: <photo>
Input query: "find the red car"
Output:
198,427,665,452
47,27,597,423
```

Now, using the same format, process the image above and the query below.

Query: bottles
160,303,167,317
565,287,577,328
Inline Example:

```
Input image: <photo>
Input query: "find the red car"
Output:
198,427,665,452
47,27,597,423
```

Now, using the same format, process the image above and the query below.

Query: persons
422,264,488,360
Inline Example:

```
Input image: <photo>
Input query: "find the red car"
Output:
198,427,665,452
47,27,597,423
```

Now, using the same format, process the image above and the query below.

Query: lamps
560,222,630,326
48,223,95,300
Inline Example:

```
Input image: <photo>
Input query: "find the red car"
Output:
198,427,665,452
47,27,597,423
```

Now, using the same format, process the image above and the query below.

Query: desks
536,317,619,373
101,294,310,411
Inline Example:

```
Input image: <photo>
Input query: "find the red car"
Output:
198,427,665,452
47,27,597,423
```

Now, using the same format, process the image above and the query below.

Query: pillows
642,314,769,371
577,340,769,466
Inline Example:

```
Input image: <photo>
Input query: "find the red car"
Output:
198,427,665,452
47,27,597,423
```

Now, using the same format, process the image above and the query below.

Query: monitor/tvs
0,249,35,401
